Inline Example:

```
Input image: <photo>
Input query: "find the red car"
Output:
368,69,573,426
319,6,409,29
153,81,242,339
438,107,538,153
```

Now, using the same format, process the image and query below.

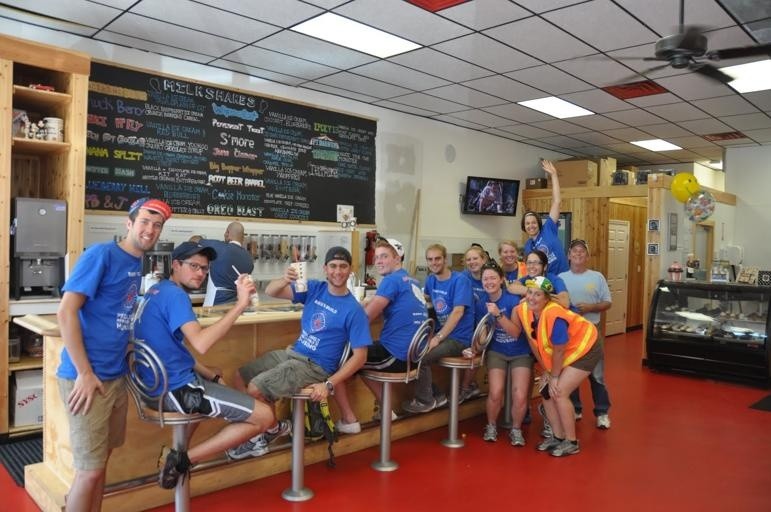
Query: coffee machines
138,239,174,297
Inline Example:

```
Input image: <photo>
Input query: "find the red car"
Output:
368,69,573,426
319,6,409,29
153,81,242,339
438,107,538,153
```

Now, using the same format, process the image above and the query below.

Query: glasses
180,259,209,273
531,320,537,339
482,264,497,268
525,260,542,266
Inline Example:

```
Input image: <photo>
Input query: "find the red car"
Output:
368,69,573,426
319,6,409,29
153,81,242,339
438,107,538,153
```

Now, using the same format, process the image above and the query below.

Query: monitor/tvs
463,176,521,216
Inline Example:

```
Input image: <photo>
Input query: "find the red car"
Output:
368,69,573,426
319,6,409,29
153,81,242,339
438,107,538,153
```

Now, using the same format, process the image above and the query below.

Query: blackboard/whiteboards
84,57,379,229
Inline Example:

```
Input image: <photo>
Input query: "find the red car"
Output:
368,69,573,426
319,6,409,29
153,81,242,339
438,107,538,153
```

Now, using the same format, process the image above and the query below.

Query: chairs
437,312,495,450
359,317,435,473
499,362,514,431
121,336,210,512
281,340,352,501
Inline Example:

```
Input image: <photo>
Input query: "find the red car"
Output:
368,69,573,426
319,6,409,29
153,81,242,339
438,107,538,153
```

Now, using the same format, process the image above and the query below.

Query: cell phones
539,382,550,400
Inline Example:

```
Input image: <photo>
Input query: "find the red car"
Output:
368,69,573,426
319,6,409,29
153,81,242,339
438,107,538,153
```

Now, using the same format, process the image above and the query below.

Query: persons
400,244,475,415
478,180,503,214
558,240,612,429
132,241,275,488
517,275,601,456
56,197,172,512
499,241,527,282
331,236,431,434
509,250,580,437
520,159,571,276
225,247,372,461
193,220,254,307
463,259,531,446
453,244,498,404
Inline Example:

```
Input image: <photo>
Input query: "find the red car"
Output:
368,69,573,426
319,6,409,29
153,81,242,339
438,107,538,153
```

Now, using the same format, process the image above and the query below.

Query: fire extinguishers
364,231,380,284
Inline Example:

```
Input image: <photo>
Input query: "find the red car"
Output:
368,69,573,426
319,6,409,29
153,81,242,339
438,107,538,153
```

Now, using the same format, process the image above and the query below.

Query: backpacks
288,398,337,444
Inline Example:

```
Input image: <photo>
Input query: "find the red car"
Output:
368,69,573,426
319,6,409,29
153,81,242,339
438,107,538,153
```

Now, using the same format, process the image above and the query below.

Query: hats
326,247,351,265
377,237,404,262
172,243,217,262
129,199,171,223
525,276,554,294
570,239,589,255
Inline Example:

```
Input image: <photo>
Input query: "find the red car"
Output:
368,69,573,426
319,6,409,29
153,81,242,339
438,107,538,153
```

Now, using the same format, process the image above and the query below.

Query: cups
290,259,310,295
231,274,260,306
42,115,64,142
341,217,359,232
351,285,366,302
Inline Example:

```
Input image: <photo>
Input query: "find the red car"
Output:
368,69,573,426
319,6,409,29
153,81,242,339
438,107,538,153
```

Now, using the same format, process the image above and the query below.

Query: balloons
672,173,701,203
686,189,717,225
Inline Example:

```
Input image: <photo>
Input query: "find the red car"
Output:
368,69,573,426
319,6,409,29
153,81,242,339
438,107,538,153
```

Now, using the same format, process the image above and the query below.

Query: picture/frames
648,218,660,232
646,243,659,255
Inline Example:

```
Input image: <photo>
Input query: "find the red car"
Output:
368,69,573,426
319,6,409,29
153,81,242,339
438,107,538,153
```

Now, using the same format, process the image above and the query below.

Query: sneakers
334,418,361,434
402,381,609,457
264,419,292,444
372,410,397,423
227,433,270,459
157,446,187,489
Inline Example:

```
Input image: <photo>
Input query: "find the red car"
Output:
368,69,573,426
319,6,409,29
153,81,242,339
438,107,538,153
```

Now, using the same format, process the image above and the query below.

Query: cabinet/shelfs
0,33,91,441
652,289,769,354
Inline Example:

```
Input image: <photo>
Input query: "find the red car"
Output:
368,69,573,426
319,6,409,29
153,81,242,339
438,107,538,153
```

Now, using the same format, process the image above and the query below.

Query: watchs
327,380,335,394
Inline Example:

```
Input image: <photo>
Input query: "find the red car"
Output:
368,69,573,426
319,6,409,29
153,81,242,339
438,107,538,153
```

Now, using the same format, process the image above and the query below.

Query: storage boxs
598,157,616,186
10,369,42,428
617,165,638,184
545,158,598,186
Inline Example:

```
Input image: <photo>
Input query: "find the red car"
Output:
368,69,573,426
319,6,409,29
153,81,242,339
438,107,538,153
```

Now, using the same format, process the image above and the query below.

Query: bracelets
496,314,504,319
213,373,220,385
437,332,445,340
550,375,559,378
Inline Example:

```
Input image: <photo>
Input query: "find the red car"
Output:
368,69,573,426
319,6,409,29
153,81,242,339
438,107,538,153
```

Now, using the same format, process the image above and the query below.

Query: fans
584,0,771,85
